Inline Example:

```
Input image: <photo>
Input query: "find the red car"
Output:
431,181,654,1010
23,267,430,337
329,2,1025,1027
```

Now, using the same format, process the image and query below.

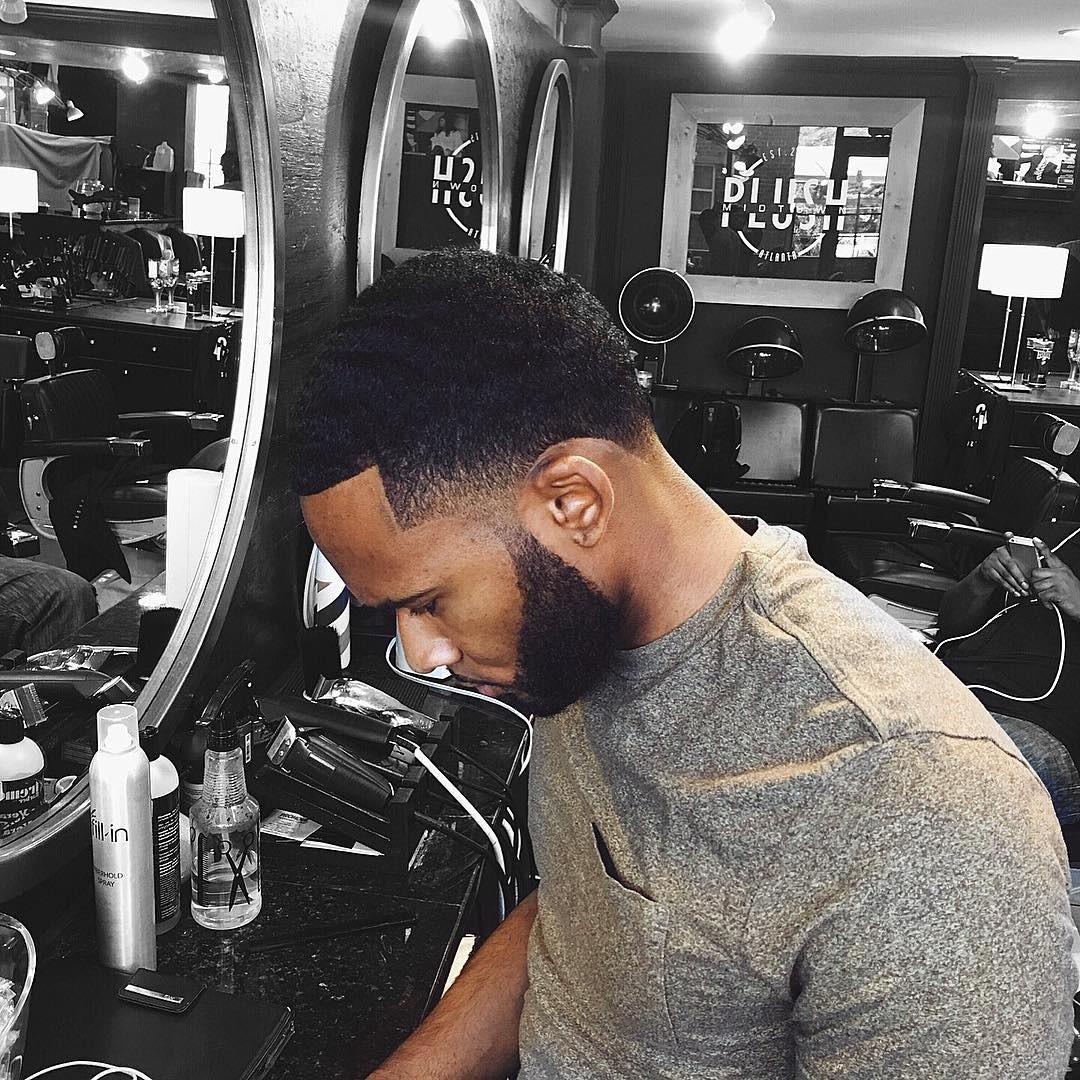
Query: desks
960,368,1080,503
0,296,242,407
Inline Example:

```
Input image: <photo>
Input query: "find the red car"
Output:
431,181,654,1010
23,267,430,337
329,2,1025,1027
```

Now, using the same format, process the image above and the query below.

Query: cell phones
1008,535,1041,582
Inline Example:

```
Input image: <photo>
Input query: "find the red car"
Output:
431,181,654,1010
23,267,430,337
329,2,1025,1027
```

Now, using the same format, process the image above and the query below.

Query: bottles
90,703,157,972
0,706,46,842
140,725,183,936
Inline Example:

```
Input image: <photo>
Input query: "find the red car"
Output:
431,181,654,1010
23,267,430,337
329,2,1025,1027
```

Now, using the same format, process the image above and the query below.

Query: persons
285,246,1080,1080
0,556,97,659
938,519,1080,825
213,149,242,191
431,116,463,157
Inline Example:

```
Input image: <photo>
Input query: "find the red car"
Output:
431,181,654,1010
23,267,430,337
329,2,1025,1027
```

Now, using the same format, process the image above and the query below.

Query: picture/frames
657,93,926,312
374,74,482,280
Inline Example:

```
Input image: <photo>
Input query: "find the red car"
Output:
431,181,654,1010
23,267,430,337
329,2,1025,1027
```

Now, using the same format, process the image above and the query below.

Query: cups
1028,337,1055,391
1022,334,1048,387
186,267,211,315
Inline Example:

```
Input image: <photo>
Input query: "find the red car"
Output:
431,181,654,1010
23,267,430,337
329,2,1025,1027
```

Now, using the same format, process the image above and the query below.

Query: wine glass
1059,329,1080,392
159,257,180,313
147,257,168,315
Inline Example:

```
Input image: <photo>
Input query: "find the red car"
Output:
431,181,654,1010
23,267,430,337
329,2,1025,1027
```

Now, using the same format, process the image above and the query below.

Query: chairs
17,326,227,542
832,413,1080,611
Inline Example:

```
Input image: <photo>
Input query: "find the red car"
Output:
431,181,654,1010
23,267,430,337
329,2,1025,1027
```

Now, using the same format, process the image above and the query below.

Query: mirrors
355,0,504,292
0,0,287,910
517,58,575,277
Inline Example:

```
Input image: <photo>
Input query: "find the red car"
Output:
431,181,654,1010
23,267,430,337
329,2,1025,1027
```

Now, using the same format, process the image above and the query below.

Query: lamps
65,99,84,123
0,0,28,24
32,80,55,106
180,183,245,323
0,166,38,239
976,242,1070,392
